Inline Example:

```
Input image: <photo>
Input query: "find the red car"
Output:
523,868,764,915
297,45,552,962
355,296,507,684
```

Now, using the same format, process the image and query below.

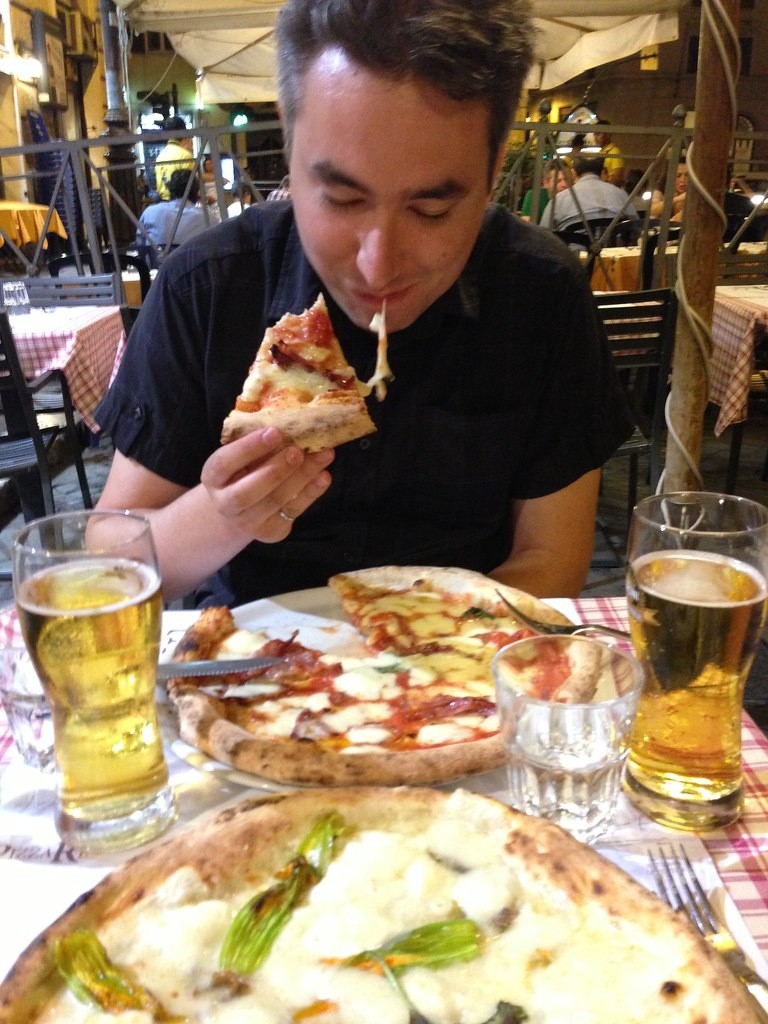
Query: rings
279,510,293,523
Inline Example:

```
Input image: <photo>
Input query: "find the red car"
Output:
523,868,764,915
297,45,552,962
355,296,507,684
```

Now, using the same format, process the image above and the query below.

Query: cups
2,281,31,306
11,509,179,857
624,492,768,832
492,634,645,845
0,604,59,774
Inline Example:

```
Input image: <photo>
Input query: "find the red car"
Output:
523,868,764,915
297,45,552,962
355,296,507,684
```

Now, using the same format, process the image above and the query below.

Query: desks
0,201,67,254
0,595,768,1024
705,284,768,511
0,304,130,447
574,240,768,355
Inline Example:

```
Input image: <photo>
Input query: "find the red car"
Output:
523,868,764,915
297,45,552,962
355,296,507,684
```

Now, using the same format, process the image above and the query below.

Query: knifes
157,659,282,680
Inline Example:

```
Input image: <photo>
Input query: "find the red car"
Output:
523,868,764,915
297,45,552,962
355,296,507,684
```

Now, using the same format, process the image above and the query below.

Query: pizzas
0,784,768,1024
168,564,597,785
222,291,395,456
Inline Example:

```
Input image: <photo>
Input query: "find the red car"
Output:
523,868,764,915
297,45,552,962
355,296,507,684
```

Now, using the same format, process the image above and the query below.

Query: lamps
550,53,661,155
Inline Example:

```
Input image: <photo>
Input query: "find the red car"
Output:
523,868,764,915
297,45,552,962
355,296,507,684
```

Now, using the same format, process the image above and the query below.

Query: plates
154,585,378,795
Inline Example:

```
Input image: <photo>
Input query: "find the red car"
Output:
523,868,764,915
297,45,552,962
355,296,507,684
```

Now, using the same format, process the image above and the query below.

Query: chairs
0,270,124,554
47,252,151,336
545,190,768,249
583,284,679,543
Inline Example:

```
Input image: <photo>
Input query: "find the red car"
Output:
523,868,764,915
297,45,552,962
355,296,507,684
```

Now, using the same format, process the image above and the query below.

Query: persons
155,116,229,205
520,120,690,249
227,181,255,220
266,175,292,202
136,169,220,245
81,0,635,596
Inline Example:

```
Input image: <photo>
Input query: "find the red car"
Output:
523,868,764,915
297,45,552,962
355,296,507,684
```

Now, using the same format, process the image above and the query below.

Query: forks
648,842,768,996
495,587,631,641
